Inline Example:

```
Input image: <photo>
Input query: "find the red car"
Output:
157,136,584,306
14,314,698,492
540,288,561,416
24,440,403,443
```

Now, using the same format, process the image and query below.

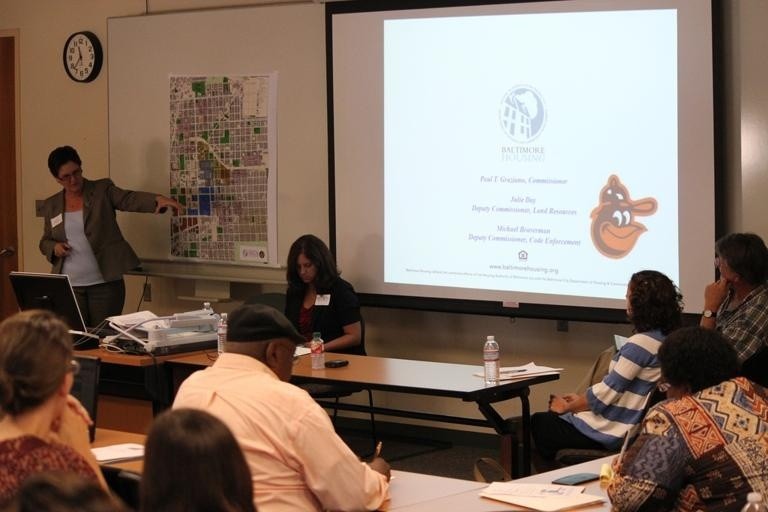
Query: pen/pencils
499,369,527,374
613,430,630,479
373,441,383,457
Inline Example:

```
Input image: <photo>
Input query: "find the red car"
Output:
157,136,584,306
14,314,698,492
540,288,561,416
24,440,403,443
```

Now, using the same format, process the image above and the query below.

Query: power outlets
143,284,152,301
557,321,568,332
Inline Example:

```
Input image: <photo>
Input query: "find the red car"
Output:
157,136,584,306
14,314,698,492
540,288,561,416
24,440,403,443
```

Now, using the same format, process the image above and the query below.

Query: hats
225,304,308,341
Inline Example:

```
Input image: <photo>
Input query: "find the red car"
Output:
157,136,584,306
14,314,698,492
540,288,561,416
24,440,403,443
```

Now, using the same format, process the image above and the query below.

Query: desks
69,327,629,512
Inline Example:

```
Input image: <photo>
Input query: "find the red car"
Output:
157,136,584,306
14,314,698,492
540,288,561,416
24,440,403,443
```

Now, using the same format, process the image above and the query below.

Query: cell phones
324,359,349,368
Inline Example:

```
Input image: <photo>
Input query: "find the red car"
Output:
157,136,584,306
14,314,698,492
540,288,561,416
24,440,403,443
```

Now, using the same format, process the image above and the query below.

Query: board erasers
132,267,143,272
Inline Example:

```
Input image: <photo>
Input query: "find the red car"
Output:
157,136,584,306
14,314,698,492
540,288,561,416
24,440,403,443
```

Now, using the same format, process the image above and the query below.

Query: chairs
333,314,376,428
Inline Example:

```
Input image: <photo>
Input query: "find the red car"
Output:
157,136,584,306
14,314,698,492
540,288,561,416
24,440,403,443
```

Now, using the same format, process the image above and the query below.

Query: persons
284,233,363,397
0,307,113,511
37,143,183,326
531,268,685,454
169,302,393,512
10,468,133,512
604,324,768,511
138,406,257,511
698,232,768,366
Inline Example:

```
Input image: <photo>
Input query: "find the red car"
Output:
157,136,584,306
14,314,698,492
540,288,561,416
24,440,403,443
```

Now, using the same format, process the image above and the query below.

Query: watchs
701,309,717,318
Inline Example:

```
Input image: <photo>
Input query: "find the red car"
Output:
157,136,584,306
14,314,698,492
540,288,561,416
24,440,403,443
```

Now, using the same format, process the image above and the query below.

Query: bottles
484,336,500,384
217,318,227,354
310,332,324,369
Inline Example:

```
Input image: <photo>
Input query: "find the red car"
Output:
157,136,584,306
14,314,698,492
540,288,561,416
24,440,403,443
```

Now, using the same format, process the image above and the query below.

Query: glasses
62,360,79,376
56,167,84,182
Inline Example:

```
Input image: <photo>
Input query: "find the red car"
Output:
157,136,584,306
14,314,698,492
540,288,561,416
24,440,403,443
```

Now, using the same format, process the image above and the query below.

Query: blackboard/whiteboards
105,0,330,285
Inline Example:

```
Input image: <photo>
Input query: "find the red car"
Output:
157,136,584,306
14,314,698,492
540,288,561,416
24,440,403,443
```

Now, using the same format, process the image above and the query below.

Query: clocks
62,30,103,82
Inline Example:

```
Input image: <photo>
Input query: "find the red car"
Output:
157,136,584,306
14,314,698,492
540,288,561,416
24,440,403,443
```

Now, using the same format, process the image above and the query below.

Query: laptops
9,271,120,338
